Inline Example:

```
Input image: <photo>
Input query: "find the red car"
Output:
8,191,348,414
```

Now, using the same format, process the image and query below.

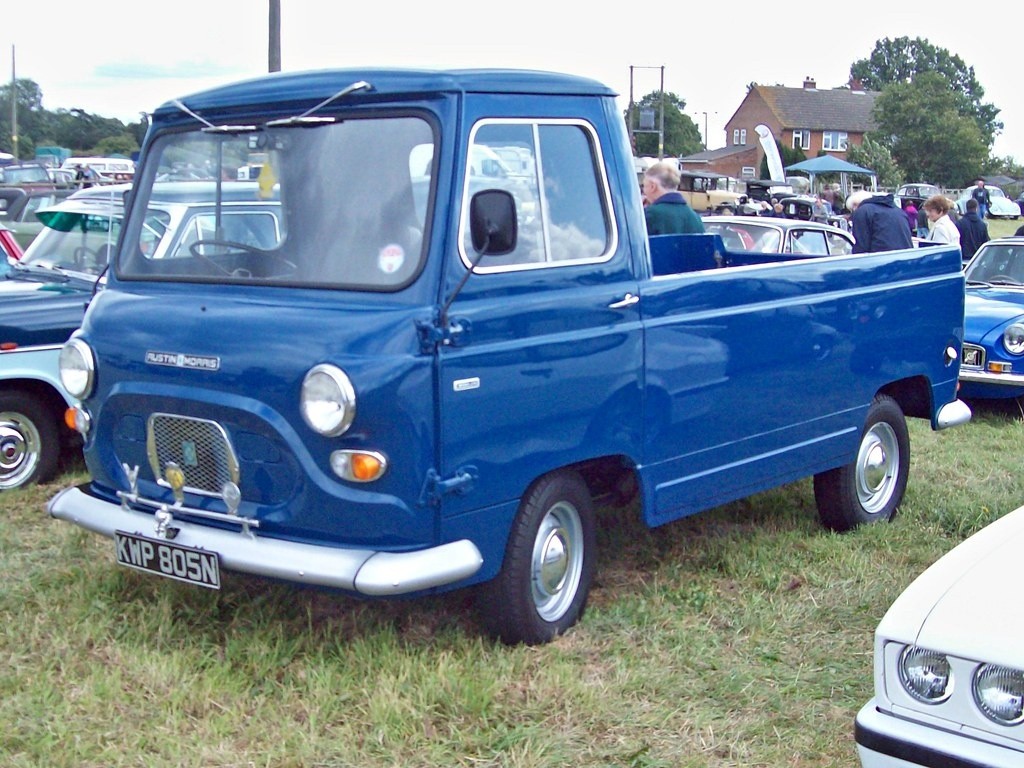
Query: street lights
702,111,709,149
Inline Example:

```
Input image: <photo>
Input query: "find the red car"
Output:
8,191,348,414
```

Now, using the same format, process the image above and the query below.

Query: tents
784,155,878,198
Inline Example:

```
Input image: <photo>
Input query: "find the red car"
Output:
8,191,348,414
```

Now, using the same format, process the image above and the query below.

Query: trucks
49,66,973,642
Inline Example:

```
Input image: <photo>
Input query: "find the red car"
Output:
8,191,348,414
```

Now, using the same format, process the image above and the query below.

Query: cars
948,235,1024,400
895,182,942,209
696,204,857,260
632,165,858,233
0,148,538,495
854,500,1024,766
954,185,1021,219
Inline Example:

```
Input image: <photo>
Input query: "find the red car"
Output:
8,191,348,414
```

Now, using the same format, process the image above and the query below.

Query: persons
846,190,914,254
820,183,843,215
808,197,829,224
644,163,706,236
972,180,991,225
74,165,94,188
734,194,786,218
905,198,934,241
926,195,961,253
955,199,990,268
